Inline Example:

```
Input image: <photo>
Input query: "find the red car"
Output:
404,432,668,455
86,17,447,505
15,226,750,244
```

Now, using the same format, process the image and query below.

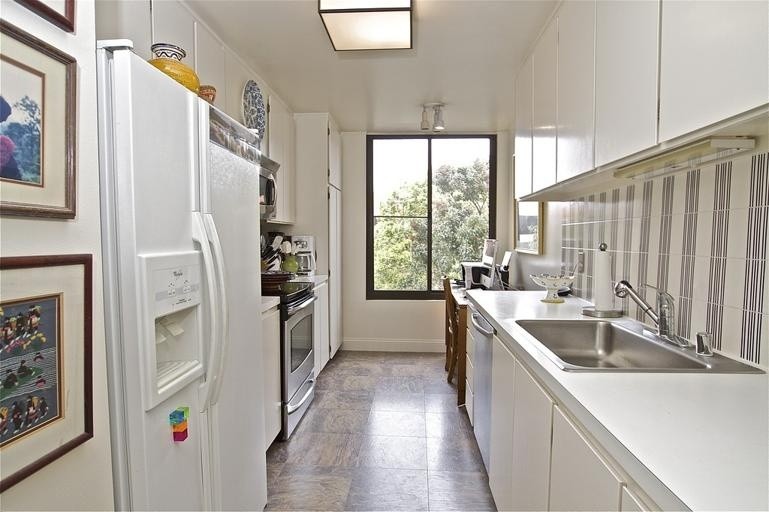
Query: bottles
147,43,216,103
283,255,298,278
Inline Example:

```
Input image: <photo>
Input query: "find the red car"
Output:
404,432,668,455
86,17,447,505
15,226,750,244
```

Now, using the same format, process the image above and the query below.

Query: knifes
263,245,279,271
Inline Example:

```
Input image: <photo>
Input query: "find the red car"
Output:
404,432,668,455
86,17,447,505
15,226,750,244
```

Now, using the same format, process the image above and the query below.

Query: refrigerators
96,39,269,512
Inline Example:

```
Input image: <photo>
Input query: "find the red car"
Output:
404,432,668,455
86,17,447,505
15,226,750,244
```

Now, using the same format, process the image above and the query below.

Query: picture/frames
0,17,79,222
0,252,96,493
510,153,545,257
13,0,80,36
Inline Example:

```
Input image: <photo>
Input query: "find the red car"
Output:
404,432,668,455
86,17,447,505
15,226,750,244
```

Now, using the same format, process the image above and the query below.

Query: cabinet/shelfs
550,403,651,512
659,3,768,144
560,4,659,183
297,186,346,361
264,93,297,225
465,302,493,476
513,1,557,198
489,335,557,512
295,109,345,189
257,310,282,452
312,278,328,375
97,0,269,156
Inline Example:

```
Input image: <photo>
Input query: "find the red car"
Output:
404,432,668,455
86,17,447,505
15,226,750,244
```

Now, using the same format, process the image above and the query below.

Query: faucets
613,279,676,339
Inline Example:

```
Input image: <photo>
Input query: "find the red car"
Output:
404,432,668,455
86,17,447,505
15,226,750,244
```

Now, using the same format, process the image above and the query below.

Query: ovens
261,281,315,442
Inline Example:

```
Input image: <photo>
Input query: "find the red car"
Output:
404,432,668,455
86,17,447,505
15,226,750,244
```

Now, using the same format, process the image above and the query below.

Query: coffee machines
292,234,316,278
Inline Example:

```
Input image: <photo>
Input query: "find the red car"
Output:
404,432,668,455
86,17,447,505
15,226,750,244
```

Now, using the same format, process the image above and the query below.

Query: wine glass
529,273,576,303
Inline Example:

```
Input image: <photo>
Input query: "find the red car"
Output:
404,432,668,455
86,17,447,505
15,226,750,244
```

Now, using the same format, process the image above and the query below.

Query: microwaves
259,153,280,220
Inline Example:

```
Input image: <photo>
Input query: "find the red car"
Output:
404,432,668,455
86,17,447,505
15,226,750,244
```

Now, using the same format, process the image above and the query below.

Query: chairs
439,275,459,382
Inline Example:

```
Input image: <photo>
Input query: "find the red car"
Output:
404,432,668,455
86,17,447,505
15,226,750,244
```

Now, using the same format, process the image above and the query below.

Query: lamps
418,102,448,135
316,0,417,51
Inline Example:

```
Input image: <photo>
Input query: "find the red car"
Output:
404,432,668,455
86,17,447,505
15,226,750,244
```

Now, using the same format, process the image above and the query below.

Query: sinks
514,319,766,374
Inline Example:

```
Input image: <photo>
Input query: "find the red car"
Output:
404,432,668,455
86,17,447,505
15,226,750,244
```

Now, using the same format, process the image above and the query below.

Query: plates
241,79,266,139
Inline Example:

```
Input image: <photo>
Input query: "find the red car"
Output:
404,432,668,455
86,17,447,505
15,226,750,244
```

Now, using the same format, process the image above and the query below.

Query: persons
0,305,48,435
1,136,22,178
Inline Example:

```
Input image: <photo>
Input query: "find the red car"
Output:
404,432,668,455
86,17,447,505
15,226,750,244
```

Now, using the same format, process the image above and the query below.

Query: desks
448,277,509,406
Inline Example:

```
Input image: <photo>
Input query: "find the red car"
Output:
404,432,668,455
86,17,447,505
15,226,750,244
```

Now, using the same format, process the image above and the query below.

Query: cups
695,334,714,356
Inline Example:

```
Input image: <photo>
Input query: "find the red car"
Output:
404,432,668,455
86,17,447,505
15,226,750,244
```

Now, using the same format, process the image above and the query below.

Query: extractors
613,136,754,181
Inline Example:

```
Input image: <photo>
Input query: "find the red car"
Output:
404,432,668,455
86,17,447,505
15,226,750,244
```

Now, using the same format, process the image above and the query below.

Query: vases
281,255,298,274
